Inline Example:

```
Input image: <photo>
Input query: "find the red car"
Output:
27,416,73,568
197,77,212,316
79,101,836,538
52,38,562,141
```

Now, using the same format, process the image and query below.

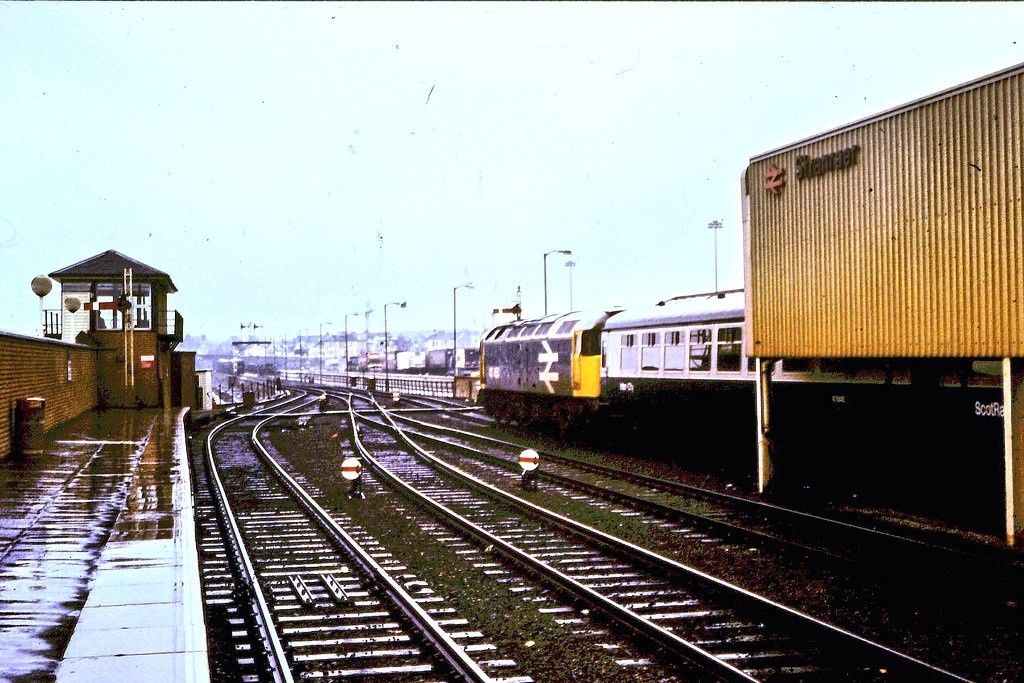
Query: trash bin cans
13,396,46,455
367,378,375,391
242,390,255,409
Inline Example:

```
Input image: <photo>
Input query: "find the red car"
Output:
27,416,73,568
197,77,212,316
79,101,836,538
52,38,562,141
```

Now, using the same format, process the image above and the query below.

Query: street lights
453,283,474,379
544,250,572,315
565,260,577,313
319,322,332,385
345,313,359,388
365,308,375,369
384,301,407,393
707,220,723,292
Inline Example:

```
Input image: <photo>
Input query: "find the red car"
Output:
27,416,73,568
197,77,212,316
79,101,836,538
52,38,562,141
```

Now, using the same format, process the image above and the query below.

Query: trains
473,282,1024,572
